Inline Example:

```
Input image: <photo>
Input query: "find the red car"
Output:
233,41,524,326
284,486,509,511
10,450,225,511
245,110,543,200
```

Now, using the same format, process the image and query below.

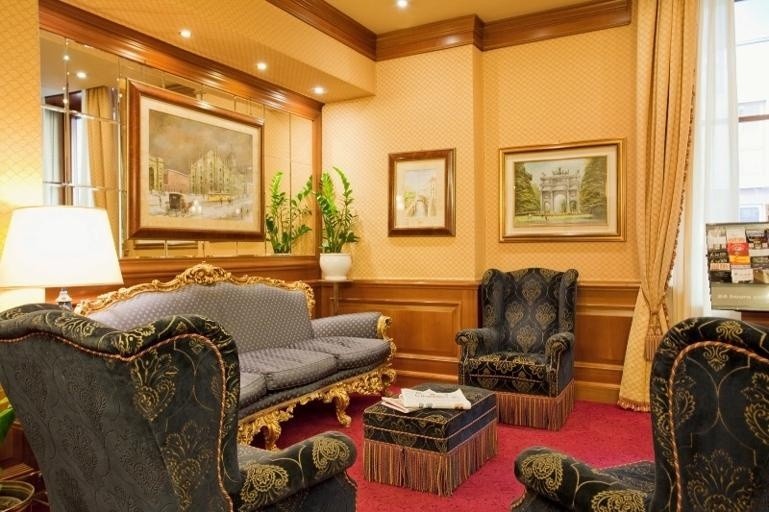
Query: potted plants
316,166,361,281
268,172,313,254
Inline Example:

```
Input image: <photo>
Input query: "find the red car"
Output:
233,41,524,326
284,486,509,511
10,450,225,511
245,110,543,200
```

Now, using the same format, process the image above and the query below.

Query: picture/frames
498,138,629,242
386,148,456,237
125,80,266,243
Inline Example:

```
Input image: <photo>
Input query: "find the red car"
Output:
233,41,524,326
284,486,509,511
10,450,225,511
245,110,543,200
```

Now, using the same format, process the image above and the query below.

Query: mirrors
40,26,313,258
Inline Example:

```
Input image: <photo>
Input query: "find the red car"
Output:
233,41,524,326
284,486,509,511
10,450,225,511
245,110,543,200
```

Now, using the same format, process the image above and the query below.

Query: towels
385,389,470,411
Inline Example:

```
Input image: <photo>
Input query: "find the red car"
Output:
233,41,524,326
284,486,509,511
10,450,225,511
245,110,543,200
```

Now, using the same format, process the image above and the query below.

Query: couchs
455,267,577,427
512,313,768,509
0,301,359,507
63,260,396,451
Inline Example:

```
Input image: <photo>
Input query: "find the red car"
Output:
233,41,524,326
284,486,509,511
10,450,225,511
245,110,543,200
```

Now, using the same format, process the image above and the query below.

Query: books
705,222,769,287
399,385,472,412
380,389,438,415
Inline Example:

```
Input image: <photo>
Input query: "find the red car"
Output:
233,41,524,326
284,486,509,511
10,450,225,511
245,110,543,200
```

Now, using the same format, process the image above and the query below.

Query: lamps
0,208,126,313
